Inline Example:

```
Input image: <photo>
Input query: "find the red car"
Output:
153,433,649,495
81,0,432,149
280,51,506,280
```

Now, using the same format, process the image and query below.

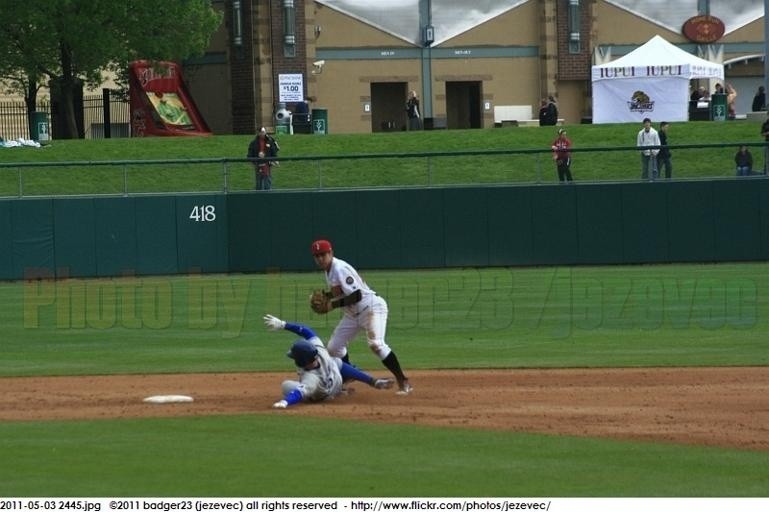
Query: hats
312,240,330,254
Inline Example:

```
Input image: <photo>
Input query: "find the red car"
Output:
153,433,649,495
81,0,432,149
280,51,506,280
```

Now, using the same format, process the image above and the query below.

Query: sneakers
396,384,413,395
375,379,394,389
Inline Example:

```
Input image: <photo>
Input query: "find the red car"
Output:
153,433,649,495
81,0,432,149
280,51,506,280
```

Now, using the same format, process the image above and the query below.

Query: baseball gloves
310,290,332,313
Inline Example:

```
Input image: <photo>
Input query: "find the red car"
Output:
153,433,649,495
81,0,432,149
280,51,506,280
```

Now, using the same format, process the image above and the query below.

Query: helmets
287,342,318,367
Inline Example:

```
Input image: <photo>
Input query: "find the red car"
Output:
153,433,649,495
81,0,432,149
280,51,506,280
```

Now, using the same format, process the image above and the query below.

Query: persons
262,314,394,409
405,90,421,130
296,98,312,121
537,82,769,184
247,127,280,190
310,239,414,396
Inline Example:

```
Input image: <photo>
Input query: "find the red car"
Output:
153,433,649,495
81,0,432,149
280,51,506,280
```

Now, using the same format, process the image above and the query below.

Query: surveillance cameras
312,60,325,68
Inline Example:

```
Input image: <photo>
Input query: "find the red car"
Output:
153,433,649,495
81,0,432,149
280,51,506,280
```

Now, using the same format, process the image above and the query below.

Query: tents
591,34,725,124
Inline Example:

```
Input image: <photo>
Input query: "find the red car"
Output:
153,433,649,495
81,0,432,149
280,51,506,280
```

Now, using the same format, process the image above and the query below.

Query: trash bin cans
30,111,50,140
311,109,327,135
712,93,727,121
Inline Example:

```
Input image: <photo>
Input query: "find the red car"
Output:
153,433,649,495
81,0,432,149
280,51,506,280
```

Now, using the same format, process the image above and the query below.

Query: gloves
273,400,287,409
265,314,283,330
